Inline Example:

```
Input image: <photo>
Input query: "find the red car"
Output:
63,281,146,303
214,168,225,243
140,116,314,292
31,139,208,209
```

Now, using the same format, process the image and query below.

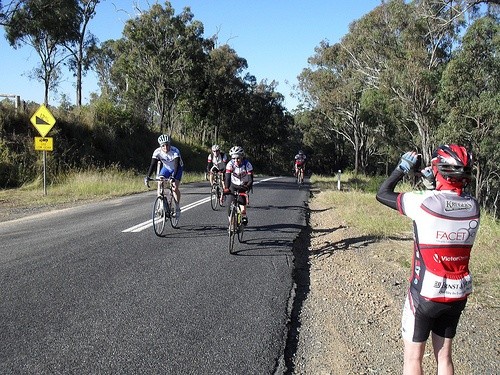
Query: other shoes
227,228,233,236
176,208,180,220
242,216,248,225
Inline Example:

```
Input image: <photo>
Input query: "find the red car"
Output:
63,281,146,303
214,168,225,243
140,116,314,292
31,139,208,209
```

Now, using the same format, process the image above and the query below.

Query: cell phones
413,154,423,174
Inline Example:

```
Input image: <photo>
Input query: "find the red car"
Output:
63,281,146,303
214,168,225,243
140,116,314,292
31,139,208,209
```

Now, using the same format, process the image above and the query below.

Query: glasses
161,143,170,147
212,150,219,153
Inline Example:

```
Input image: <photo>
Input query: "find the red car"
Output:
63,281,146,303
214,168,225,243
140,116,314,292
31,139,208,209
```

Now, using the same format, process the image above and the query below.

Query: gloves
145,176,150,181
224,188,230,194
239,187,247,193
170,177,175,183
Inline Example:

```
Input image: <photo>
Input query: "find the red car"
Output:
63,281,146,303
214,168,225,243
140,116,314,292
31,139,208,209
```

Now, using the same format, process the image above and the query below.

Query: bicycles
146,175,181,237
296,166,304,190
204,170,227,211
222,189,247,254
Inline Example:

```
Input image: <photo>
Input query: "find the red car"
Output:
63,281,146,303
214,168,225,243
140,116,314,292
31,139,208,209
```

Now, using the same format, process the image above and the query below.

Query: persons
294,150,307,184
207,145,227,205
224,146,253,237
145,134,183,219
375,144,480,375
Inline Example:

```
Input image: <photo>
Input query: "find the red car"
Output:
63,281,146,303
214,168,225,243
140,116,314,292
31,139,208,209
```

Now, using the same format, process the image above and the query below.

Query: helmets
298,151,304,155
212,144,220,151
158,135,171,144
431,144,474,183
229,147,246,158
